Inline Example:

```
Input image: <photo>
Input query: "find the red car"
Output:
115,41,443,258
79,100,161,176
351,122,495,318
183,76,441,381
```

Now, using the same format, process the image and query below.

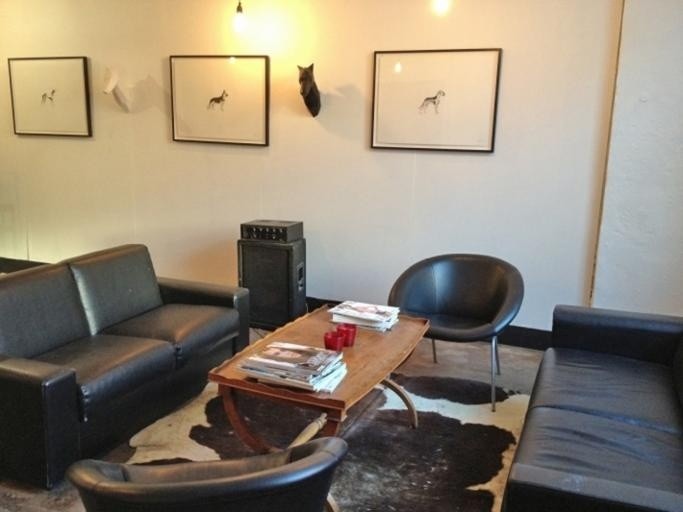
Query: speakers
236,239,307,331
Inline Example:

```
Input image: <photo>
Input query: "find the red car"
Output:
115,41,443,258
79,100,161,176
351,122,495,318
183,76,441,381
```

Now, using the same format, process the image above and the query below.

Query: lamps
231,1,247,33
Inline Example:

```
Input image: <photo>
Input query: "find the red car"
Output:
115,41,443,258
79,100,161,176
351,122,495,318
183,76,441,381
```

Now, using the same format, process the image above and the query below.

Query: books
325,299,400,334
233,340,348,397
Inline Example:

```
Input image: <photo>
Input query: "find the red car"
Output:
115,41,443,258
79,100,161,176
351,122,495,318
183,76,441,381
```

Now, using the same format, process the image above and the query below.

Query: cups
323,323,356,351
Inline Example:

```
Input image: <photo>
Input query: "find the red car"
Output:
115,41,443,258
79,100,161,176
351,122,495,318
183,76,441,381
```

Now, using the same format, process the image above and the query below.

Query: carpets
125,370,531,512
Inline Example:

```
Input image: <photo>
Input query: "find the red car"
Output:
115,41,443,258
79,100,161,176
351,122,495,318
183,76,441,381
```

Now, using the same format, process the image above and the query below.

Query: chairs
67,436,348,512
388,254,523,413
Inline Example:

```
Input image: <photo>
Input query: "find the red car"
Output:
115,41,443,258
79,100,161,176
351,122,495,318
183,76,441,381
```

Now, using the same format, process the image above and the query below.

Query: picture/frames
371,48,502,153
8,57,92,137
169,55,270,147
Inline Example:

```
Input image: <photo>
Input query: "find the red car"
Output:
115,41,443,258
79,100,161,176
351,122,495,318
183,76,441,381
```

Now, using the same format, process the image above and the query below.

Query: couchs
0,243,249,490
501,305,683,511
